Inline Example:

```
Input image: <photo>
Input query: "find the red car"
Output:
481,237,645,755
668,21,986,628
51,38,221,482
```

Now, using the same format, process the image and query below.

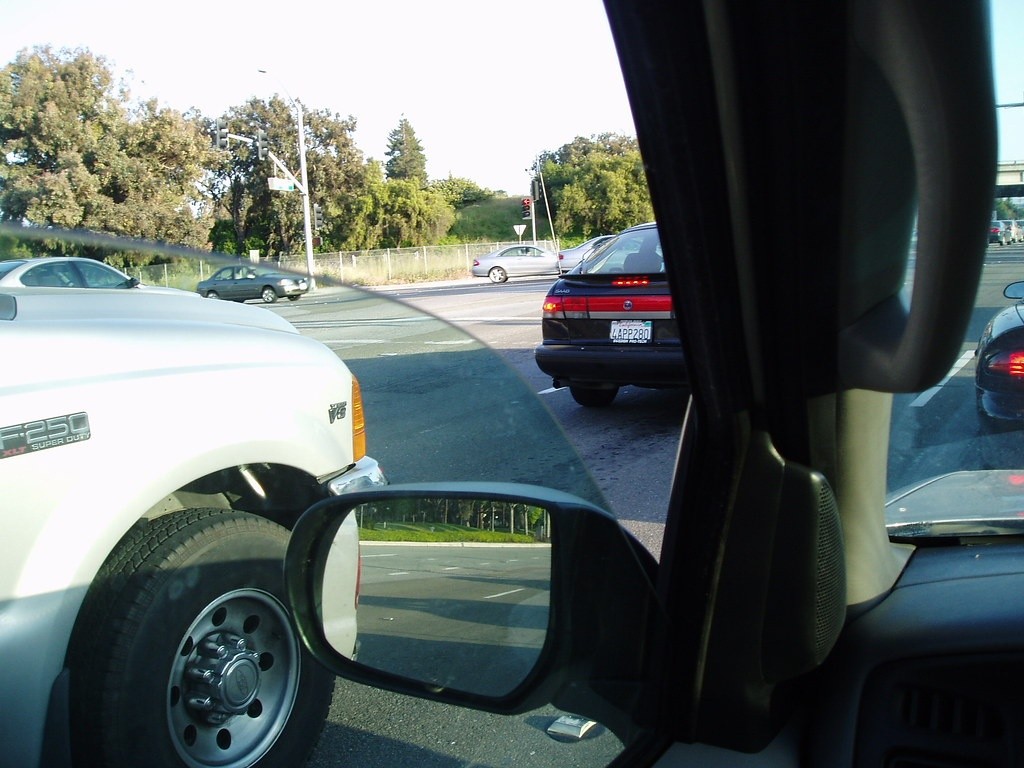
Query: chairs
623,253,662,272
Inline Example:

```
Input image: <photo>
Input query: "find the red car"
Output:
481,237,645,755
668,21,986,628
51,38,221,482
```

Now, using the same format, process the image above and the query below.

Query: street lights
258,66,316,289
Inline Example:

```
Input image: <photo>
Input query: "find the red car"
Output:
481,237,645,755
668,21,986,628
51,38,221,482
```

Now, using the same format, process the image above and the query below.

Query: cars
0,287,392,768
974,281,1024,424
0,257,202,296
196,265,315,303
556,235,665,273
1001,220,1024,243
534,222,689,406
471,245,559,283
989,221,1013,246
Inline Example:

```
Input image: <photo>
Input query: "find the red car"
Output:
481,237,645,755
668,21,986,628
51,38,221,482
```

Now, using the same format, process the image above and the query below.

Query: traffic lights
216,118,228,151
521,196,532,220
258,130,269,161
